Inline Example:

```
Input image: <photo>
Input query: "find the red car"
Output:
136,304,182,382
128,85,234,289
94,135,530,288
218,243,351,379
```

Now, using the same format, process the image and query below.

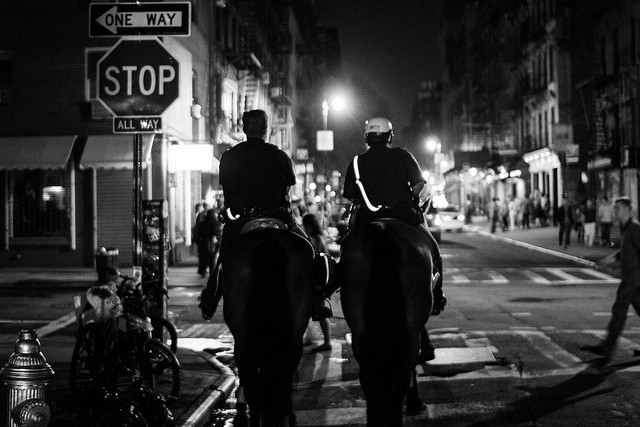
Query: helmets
363,118,393,136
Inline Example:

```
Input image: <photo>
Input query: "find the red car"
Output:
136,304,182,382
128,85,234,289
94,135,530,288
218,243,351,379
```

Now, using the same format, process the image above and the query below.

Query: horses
221,203,316,427
337,200,436,423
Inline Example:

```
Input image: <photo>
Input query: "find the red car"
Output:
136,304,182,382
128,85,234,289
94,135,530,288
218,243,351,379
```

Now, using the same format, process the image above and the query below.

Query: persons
599,196,615,247
541,193,551,226
531,187,541,228
558,198,573,246
301,213,331,355
201,109,334,320
193,201,206,276
521,194,531,229
501,202,509,229
339,117,447,335
575,203,584,241
509,199,521,230
206,197,224,277
593,198,640,354
584,200,597,246
486,198,499,232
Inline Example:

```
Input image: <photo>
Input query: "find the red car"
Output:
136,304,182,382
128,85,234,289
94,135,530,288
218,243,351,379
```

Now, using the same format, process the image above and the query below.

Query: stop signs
96,37,182,116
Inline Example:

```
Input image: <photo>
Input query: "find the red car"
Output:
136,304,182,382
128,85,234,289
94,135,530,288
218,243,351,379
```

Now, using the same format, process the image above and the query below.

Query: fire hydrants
0,325,55,426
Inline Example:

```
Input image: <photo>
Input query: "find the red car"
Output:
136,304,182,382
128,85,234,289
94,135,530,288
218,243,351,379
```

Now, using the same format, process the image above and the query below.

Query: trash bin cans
96,247,120,281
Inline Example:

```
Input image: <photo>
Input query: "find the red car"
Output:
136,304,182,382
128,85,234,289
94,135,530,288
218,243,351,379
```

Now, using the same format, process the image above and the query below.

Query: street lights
322,92,349,217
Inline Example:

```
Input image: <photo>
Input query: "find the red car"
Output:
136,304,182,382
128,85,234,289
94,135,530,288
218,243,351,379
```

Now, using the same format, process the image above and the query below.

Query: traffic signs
89,1,191,36
113,115,163,133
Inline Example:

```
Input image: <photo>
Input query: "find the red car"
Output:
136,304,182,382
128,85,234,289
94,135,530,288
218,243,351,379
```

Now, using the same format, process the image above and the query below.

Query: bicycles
71,265,180,425
